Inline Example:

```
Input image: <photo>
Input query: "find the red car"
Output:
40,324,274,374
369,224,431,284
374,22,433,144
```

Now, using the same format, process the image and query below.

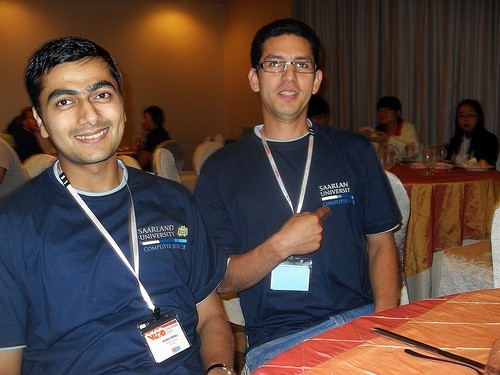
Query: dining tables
380,160,500,303
244,287,500,375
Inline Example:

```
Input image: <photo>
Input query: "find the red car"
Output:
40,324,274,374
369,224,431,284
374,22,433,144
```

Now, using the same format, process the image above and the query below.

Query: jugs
377,150,395,170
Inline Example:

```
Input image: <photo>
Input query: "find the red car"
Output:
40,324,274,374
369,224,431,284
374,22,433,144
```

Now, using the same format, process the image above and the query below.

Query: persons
1,116,20,146
134,105,172,171
306,96,333,126
0,36,239,375
195,17,410,375
14,105,48,160
359,95,421,161
443,98,499,169
0,136,31,197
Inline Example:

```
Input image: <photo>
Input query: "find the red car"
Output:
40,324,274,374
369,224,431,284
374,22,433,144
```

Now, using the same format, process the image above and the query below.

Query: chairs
429,198,500,298
380,168,411,307
22,133,227,185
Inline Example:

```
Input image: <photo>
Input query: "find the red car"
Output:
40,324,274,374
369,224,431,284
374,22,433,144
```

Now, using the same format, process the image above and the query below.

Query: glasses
258,60,317,73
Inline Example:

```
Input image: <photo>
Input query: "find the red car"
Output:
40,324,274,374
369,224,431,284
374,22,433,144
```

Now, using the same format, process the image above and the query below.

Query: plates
457,165,496,172
427,162,455,169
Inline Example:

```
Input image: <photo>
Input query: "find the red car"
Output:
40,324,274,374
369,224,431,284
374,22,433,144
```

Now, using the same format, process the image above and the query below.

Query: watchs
206,363,237,375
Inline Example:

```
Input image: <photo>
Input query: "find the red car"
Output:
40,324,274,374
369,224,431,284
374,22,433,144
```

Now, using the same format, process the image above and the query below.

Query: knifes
372,327,500,373
